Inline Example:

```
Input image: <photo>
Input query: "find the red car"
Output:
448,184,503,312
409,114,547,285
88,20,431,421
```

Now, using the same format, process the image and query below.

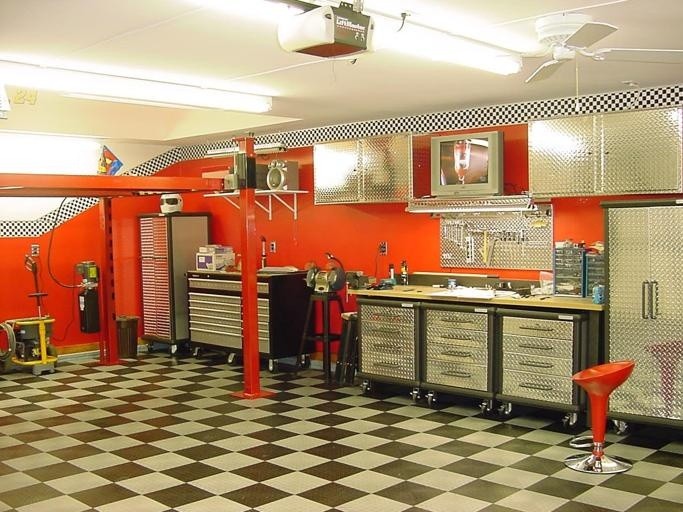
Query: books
429,288,496,300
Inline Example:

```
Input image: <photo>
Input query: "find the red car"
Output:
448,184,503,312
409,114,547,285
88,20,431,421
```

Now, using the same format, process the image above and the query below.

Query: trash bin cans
116,316,141,359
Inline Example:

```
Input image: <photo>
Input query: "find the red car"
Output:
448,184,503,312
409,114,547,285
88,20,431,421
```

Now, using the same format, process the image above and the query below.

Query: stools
564,360,635,474
642,341,683,419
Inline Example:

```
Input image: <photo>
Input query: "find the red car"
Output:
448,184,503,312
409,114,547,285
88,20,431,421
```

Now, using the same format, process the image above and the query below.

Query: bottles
400,260,409,285
592,282,603,304
388,264,395,278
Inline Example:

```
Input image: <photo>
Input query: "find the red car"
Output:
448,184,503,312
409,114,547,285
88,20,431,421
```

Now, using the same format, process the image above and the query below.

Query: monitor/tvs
431,131,503,197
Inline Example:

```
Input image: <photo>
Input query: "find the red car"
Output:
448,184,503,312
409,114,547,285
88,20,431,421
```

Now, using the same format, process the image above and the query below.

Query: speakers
267,159,299,190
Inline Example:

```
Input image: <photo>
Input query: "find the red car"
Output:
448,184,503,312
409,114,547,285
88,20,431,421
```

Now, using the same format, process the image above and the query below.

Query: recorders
306,262,345,295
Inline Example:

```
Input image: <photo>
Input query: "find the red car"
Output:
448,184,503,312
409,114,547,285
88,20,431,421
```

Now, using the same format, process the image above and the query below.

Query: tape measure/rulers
477,238,495,268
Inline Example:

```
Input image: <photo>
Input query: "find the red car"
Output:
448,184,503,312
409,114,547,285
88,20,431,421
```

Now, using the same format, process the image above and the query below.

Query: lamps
1,60,273,115
520,13,683,84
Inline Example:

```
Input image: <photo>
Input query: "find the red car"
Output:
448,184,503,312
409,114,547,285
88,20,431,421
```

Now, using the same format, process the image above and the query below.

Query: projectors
276,6,375,61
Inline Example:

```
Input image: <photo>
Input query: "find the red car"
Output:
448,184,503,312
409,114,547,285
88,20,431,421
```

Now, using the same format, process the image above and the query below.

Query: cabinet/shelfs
313,132,440,205
598,201,683,435
356,298,588,429
137,213,209,354
184,271,314,372
528,105,682,198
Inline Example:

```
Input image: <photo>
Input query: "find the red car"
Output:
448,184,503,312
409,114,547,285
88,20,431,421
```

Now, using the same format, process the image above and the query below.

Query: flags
96,146,123,177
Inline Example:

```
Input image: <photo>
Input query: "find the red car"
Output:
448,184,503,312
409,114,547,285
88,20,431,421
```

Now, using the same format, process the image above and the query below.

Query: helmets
157,192,184,214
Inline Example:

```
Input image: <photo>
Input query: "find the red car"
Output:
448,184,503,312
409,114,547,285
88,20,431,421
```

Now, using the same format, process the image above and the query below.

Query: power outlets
379,241,387,256
